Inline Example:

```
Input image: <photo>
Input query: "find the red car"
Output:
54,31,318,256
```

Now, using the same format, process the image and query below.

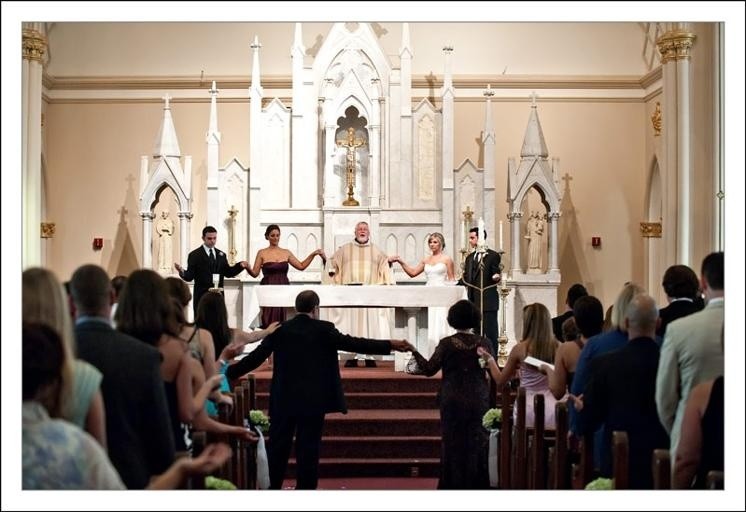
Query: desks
254,285,465,308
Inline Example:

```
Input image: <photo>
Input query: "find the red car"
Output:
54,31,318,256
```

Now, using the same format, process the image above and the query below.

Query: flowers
480,405,509,432
584,478,615,490
202,475,240,489
243,408,274,432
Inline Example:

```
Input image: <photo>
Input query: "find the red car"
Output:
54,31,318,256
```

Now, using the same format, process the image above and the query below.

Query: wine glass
212,273,220,288
328,266,337,286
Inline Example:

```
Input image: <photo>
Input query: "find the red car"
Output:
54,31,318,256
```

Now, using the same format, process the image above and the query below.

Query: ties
210,249,214,261
473,253,479,278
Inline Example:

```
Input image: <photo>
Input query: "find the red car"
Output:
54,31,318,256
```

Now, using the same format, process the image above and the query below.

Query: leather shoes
344,359,358,367
365,361,376,367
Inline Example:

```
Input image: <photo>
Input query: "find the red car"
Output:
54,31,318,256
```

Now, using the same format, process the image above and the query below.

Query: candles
212,274,219,284
500,221,502,249
461,221,464,249
479,217,484,242
502,273,507,281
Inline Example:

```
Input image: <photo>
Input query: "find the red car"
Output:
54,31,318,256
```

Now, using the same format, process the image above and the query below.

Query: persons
457,227,502,360
395,231,455,361
478,248,724,490
22,264,283,489
525,209,546,272
319,222,398,367
176,226,245,326
405,299,494,487
155,209,176,272
226,289,411,490
241,225,319,369
339,139,368,191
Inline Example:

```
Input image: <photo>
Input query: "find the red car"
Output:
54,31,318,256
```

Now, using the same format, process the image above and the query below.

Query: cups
388,268,398,284
